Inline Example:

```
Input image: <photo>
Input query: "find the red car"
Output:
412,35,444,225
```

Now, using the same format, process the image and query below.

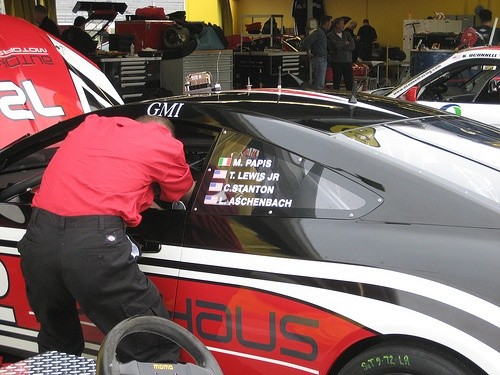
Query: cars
354,45,500,132
0,13,500,375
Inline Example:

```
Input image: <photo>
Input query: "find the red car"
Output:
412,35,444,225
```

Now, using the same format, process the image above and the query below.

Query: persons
17,111,197,364
60,15,96,61
176,164,245,254
99,24,110,44
302,8,500,91
32,5,59,38
